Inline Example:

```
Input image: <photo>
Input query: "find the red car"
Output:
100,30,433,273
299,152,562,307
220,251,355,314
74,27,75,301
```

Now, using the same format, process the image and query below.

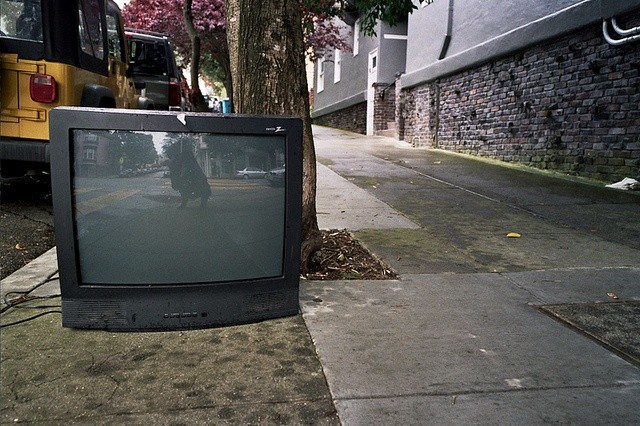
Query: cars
266,163,286,187
204,94,222,112
232,166,267,180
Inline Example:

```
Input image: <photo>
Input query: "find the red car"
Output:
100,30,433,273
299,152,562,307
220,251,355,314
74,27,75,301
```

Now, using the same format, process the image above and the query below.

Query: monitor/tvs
48,106,304,333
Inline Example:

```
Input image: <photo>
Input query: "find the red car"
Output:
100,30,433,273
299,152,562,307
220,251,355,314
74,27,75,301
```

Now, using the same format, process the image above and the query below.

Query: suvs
1,0,157,192
78,25,192,113
